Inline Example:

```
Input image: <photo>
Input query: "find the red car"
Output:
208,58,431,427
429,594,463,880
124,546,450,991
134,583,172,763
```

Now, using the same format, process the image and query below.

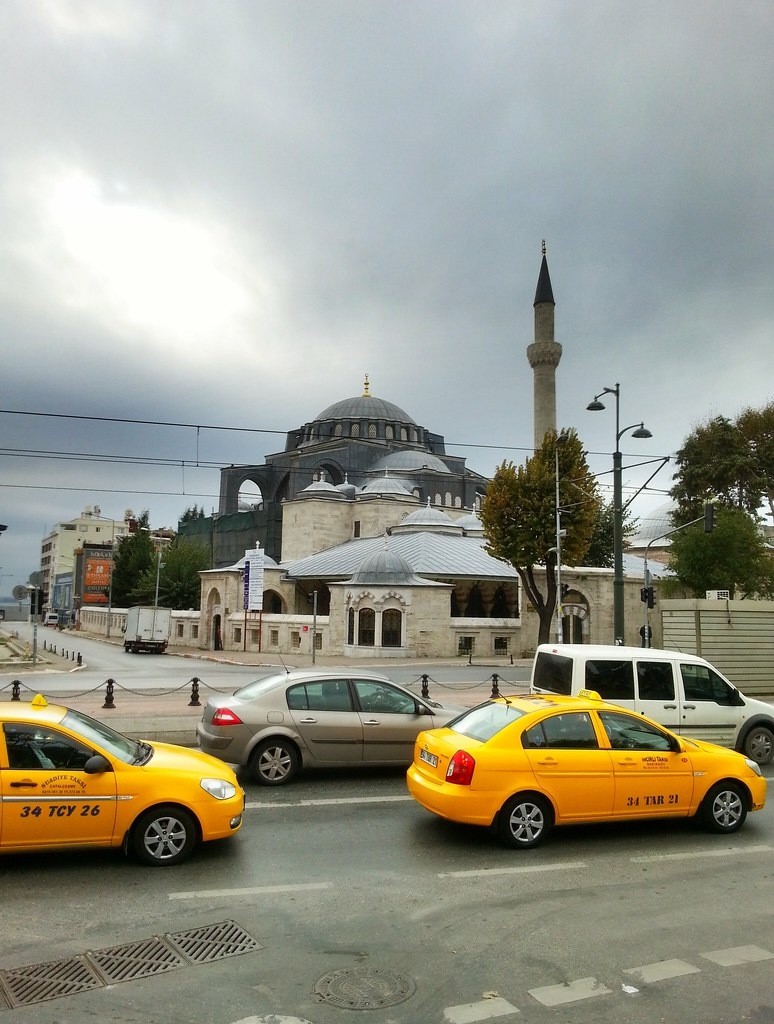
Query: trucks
121,606,172,653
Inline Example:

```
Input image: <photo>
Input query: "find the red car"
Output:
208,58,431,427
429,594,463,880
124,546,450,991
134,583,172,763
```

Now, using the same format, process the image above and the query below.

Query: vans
528,643,774,767
44,613,59,626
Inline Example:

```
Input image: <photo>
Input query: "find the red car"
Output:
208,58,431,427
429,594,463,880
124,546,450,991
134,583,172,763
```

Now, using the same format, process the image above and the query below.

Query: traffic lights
648,586,657,609
641,587,648,603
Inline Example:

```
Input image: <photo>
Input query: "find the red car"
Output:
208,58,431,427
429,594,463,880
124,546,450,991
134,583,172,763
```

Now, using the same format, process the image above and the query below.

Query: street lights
587,381,654,645
140,527,163,607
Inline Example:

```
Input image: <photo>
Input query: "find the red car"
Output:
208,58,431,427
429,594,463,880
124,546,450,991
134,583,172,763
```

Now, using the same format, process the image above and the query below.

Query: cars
0,692,247,868
405,688,767,850
195,665,493,788
